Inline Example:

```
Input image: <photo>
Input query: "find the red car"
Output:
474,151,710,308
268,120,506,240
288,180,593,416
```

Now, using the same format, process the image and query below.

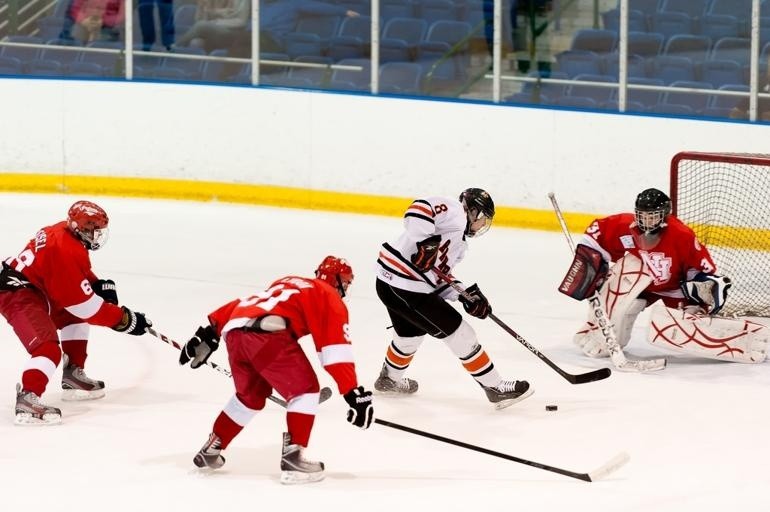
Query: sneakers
62,353,104,390
374,362,418,393
484,380,530,402
16,383,61,419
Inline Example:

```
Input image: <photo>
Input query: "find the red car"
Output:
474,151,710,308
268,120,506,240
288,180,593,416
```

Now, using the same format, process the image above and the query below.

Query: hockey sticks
372,417,629,481
145,328,332,408
431,265,612,384
548,193,667,371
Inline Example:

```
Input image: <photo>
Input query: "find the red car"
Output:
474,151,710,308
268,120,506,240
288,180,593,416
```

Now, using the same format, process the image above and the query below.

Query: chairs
0,0,769,120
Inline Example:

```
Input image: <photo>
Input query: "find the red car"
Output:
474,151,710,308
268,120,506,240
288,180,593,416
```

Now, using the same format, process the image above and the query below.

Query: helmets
635,188,670,233
66,201,109,251
460,188,495,237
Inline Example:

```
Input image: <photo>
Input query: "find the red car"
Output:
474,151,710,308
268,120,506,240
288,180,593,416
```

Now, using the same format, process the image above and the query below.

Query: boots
193,432,225,467
280,432,324,472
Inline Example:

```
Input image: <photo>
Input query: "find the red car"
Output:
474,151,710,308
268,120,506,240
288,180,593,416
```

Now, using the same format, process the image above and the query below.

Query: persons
0,199,153,425
56,0,125,46
558,188,733,357
179,256,373,484
373,189,534,410
171,0,250,54
481,1,561,82
136,2,176,53
222,2,361,81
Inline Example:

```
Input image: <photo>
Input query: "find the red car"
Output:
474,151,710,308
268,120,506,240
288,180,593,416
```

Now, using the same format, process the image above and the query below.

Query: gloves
179,326,219,368
459,283,492,319
411,235,441,273
343,387,373,428
93,280,153,335
